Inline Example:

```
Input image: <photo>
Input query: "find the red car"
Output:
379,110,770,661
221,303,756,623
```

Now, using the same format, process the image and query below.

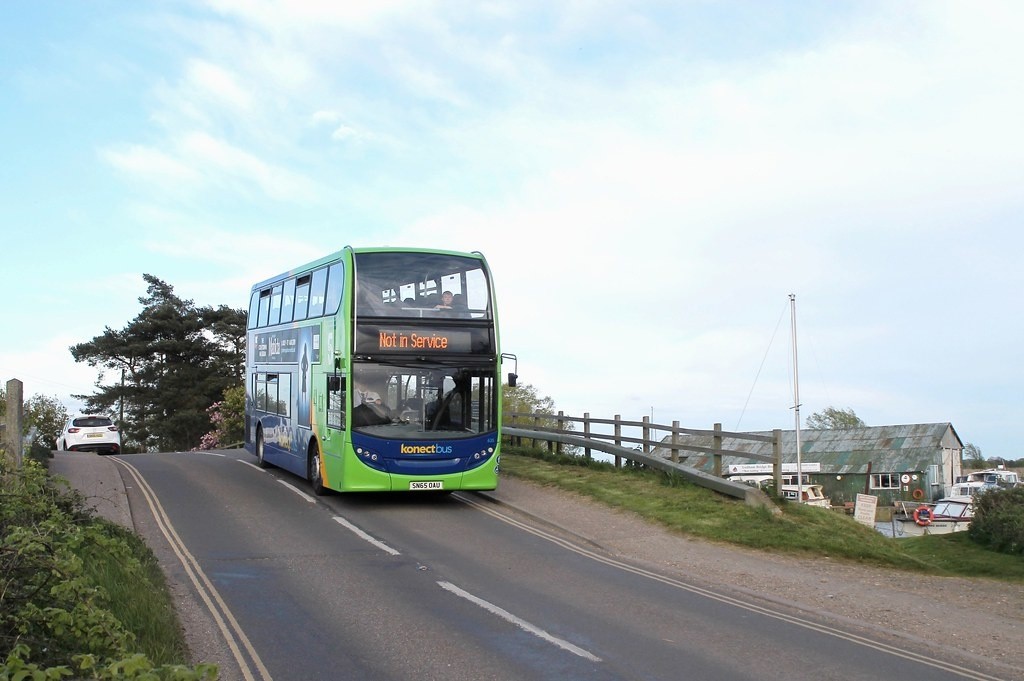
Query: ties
361,393,366,403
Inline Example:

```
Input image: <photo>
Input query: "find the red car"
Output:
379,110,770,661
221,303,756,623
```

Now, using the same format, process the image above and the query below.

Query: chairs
271,301,322,322
425,294,443,305
414,296,426,305
453,294,471,318
402,298,416,307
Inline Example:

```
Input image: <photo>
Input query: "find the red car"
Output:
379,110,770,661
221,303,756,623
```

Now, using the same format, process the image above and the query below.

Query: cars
54,415,120,455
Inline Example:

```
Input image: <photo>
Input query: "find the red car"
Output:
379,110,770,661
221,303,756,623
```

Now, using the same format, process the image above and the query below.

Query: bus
247,246,519,495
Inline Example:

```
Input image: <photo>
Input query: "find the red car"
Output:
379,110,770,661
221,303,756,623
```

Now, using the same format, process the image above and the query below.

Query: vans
764,483,824,506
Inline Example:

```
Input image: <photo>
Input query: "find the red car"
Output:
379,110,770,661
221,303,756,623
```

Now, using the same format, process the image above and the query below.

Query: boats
891,459,1019,536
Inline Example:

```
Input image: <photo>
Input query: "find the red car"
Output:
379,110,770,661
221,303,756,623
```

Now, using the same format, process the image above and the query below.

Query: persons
300,342,308,404
434,291,456,310
353,376,381,407
